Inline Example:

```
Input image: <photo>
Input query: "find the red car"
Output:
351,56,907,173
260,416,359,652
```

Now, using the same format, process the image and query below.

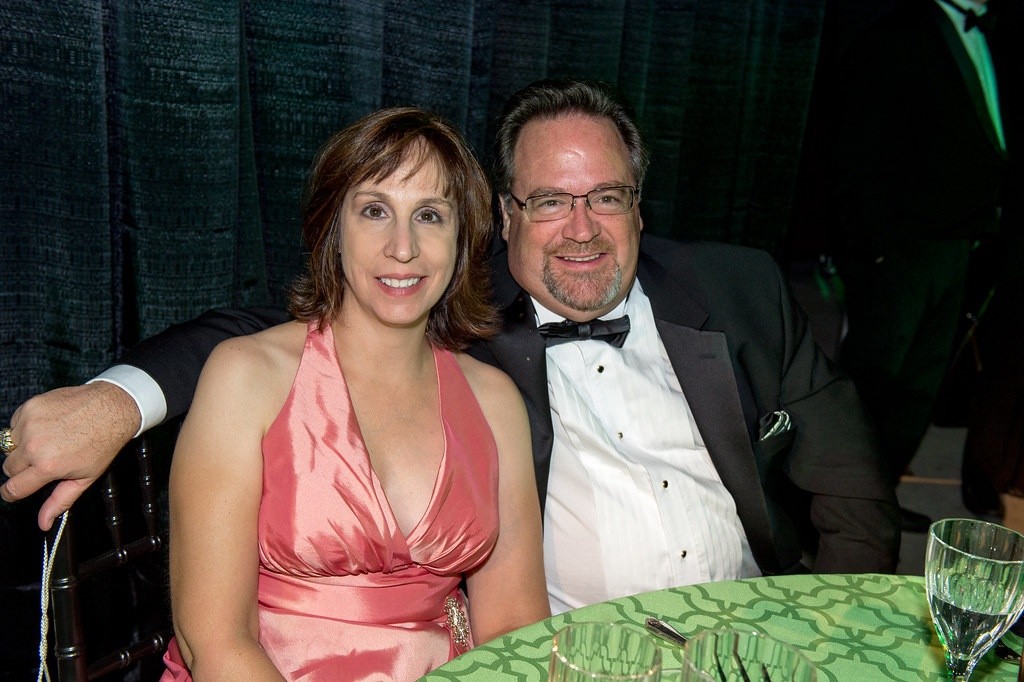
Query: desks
413,574,1024,682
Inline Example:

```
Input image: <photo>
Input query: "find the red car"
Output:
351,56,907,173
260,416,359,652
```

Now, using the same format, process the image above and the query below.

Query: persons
160,106,553,682
0,74,903,613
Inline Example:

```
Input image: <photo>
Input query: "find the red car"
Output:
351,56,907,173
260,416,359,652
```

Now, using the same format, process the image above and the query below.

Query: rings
0,427,17,455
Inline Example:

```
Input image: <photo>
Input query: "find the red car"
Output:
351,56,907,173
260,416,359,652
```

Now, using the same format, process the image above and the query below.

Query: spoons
994,637,1021,664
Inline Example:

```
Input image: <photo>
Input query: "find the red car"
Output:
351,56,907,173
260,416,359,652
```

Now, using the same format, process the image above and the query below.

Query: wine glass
924,518,1024,682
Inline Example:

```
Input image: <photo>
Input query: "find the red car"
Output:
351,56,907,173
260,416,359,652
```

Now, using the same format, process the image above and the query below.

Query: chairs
42,412,189,682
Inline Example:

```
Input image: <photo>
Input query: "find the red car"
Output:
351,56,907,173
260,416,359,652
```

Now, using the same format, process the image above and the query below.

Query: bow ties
538,315,631,348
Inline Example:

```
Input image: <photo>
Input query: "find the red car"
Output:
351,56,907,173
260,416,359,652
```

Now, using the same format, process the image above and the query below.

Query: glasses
510,184,637,223
